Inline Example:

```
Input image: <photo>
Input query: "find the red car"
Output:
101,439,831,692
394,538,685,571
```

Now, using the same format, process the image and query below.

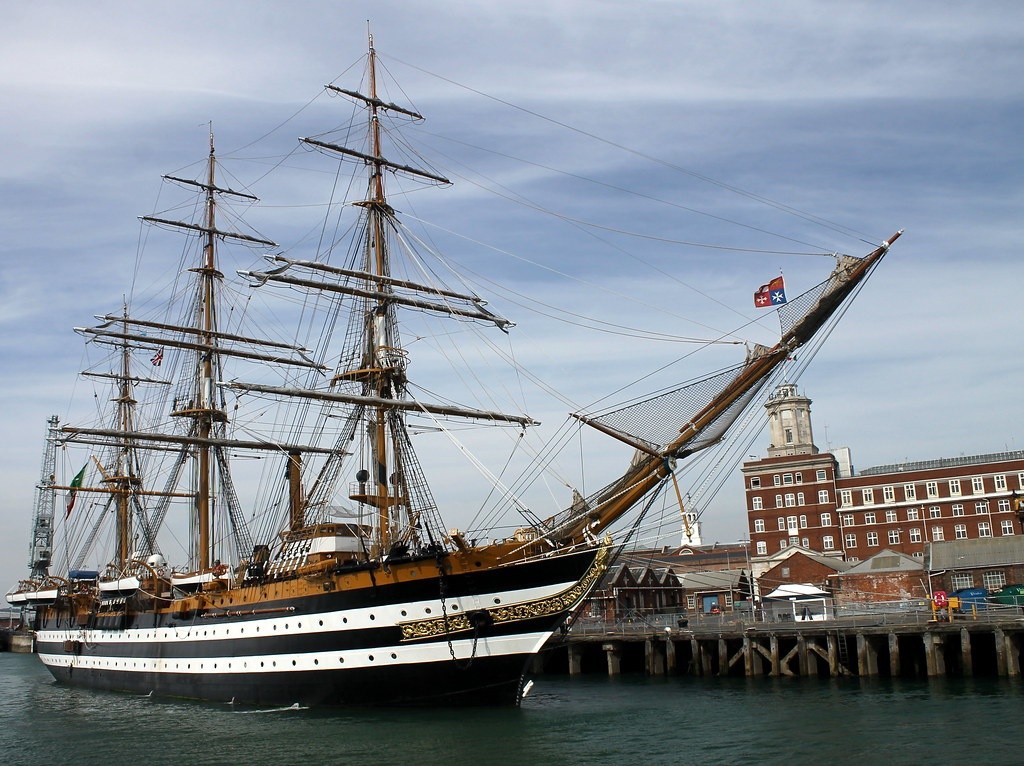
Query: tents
762,583,834,622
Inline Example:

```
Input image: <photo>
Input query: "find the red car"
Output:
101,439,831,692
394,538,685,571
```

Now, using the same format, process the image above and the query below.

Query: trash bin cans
947,587,987,613
677,619,688,630
992,584,1024,604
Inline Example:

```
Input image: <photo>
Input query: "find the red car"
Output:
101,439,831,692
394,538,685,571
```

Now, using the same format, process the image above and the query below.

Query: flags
150,346,164,366
754,276,788,308
70,465,87,496
65,490,77,521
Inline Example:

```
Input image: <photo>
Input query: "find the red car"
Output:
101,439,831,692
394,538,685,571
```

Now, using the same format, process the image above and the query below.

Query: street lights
724,549,734,611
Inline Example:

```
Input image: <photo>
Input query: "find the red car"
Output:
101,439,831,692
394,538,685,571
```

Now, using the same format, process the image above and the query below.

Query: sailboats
4,11,902,713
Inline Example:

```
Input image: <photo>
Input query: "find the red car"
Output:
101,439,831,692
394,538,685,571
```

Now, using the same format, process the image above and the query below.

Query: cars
710,604,723,615
